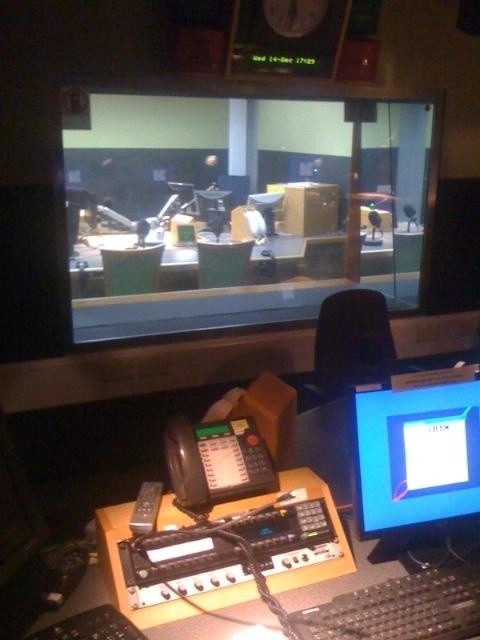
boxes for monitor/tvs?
[344,361,480,572]
[46,61,447,355]
[64,175,292,236]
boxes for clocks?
[224,0,353,82]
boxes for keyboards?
[281,565,480,640]
[23,603,149,640]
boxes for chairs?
[101,234,367,296]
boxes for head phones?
[260,249,276,276]
[77,261,89,287]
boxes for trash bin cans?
[166,414,280,511]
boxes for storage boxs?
[281,180,339,236]
[234,366,299,473]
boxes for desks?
[1,467,479,639]
[68,229,392,303]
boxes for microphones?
[136,221,150,243]
[368,211,383,237]
[403,203,417,227]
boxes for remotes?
[32,548,93,611]
[129,481,164,533]
